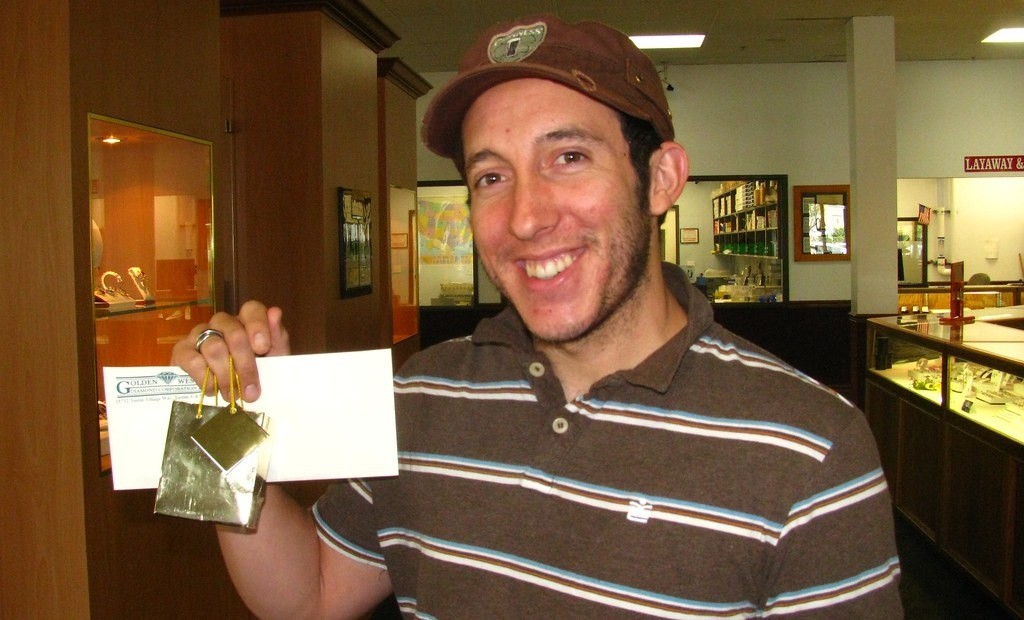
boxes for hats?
[420,13,674,159]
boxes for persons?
[171,16,907,620]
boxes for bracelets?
[101,269,149,291]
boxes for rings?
[196,329,224,353]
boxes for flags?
[918,204,930,224]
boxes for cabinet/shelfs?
[94,298,215,473]
[712,178,780,262]
